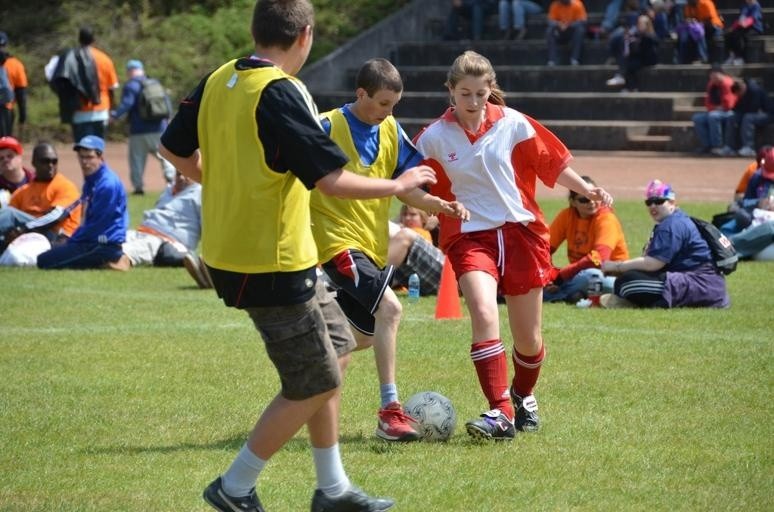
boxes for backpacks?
[135,78,169,121]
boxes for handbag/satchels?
[690,216,739,275]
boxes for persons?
[37,137,127,270]
[544,179,628,304]
[381,204,459,296]
[65,25,119,140]
[1,139,81,268]
[111,58,175,195]
[499,0,774,154]
[1,32,30,139]
[159,3,438,511]
[310,57,470,438]
[183,252,215,289]
[411,49,613,438]
[111,166,203,269]
[715,139,774,264]
[600,180,728,309]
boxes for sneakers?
[184,253,208,289]
[465,407,515,440]
[511,386,540,431]
[608,78,624,85]
[376,402,424,441]
[599,293,633,309]
[198,255,215,288]
[310,487,395,511]
[203,477,263,512]
[712,146,757,156]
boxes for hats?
[0,137,24,155]
[646,180,675,201]
[73,135,104,152]
[762,148,774,179]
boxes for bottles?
[588,273,602,305]
[408,269,420,301]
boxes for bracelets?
[616,260,623,274]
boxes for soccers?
[403,393,455,441]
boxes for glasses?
[645,198,667,207]
[576,197,590,204]
